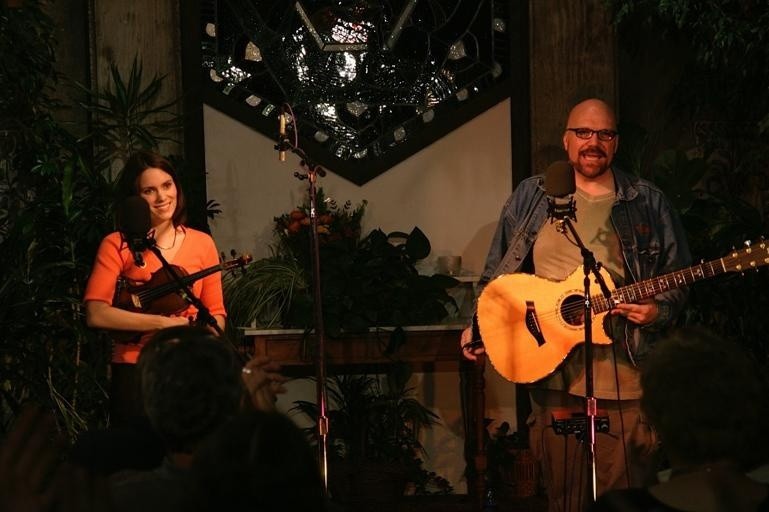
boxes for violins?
[110,254,253,344]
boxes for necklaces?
[153,226,176,250]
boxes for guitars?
[476,233,769,384]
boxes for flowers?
[272,186,368,270]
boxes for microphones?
[119,194,151,254]
[545,159,577,232]
[277,103,290,162]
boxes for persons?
[462,98,692,512]
[584,327,769,512]
[2,324,327,512]
[83,155,227,474]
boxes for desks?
[243,324,495,511]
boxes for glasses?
[567,127,618,141]
